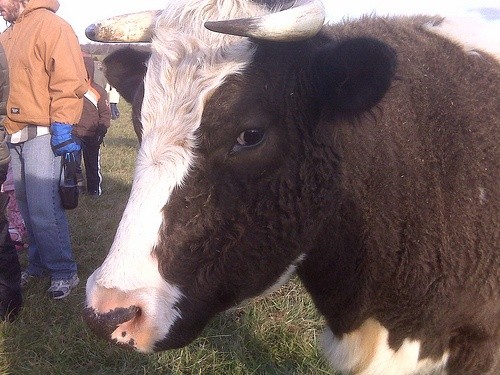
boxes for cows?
[81,0,500,375]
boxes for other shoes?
[88,189,102,196]
[76,180,86,195]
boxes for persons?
[0,43,23,321]
[0,0,89,300]
[71,57,110,198]
[93,56,120,120]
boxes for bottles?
[58,152,79,209]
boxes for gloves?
[109,102,120,120]
[0,165,10,183]
[50,123,81,156]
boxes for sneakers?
[45,273,79,300]
[19,272,37,288]
